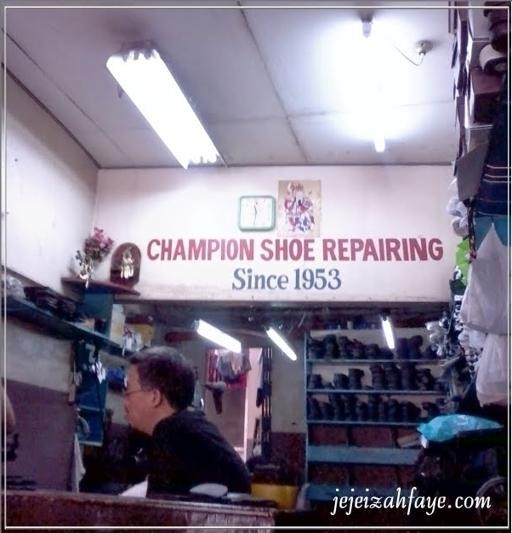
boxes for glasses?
[121,388,152,398]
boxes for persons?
[0,381,16,432]
[124,346,251,506]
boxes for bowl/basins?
[250,481,299,509]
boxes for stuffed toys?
[458,332,482,371]
[425,317,447,357]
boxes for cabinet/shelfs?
[302,329,459,505]
[75,405,106,449]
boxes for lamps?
[105,39,221,172]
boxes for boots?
[307,335,441,421]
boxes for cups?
[189,482,227,499]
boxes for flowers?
[77,225,116,288]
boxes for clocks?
[238,195,276,232]
